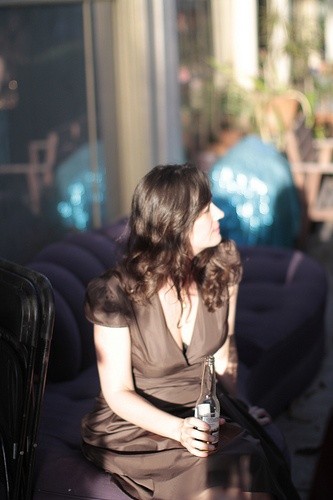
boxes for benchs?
[286,117,333,242]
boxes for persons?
[80,164,301,500]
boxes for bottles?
[194,356,220,453]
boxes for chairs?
[0,258,56,500]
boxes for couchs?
[30,216,329,500]
